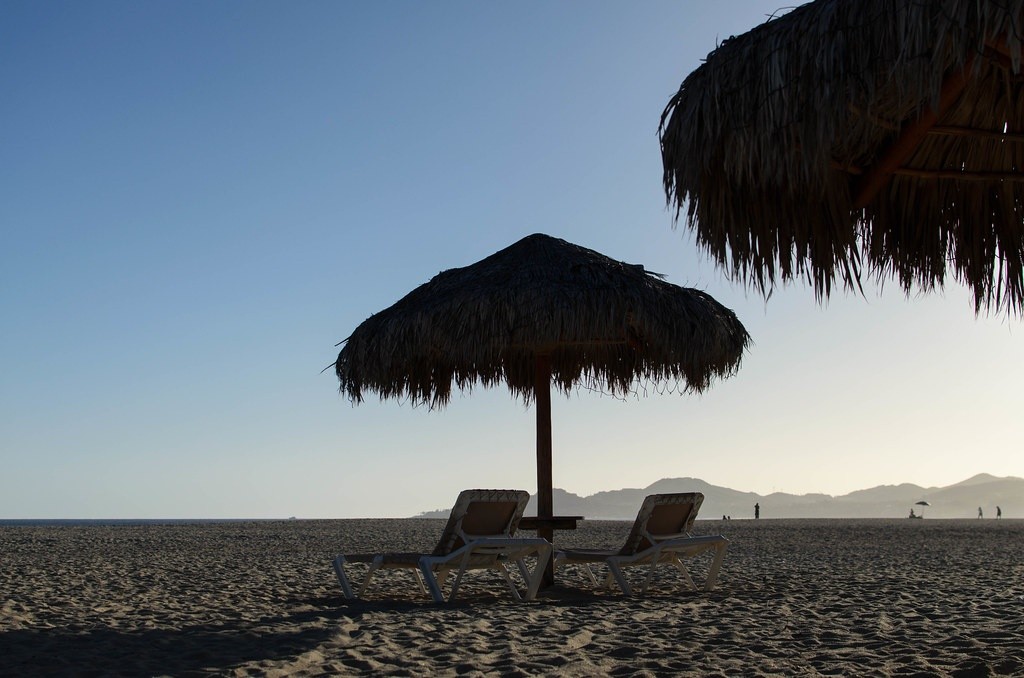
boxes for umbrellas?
[317,231,755,603]
[656,0,1023,322]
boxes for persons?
[995,506,1002,519]
[722,515,726,520]
[977,507,983,519]
[909,509,914,516]
[754,503,760,519]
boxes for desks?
[513,515,586,596]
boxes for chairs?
[527,491,730,596]
[333,489,528,603]
